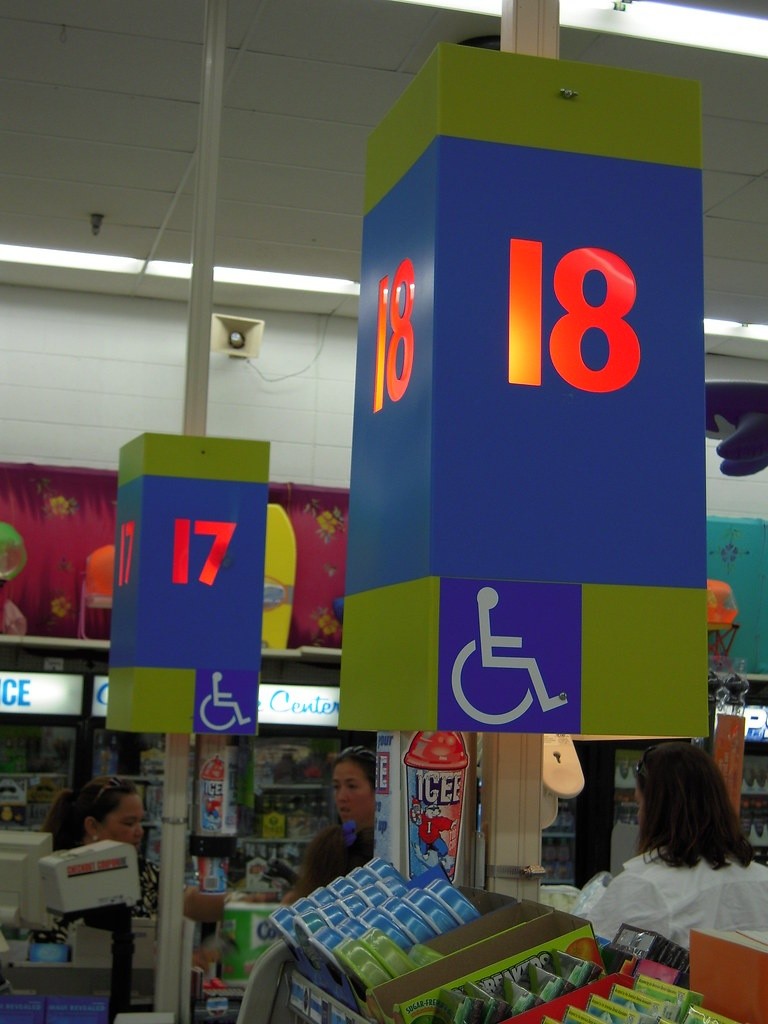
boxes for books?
[689,928,768,1024]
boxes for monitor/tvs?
[0,830,55,931]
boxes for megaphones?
[210,313,264,359]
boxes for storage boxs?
[280,886,640,1024]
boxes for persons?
[34,776,275,1024]
[583,741,768,948]
[282,747,377,904]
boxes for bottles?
[254,793,329,839]
